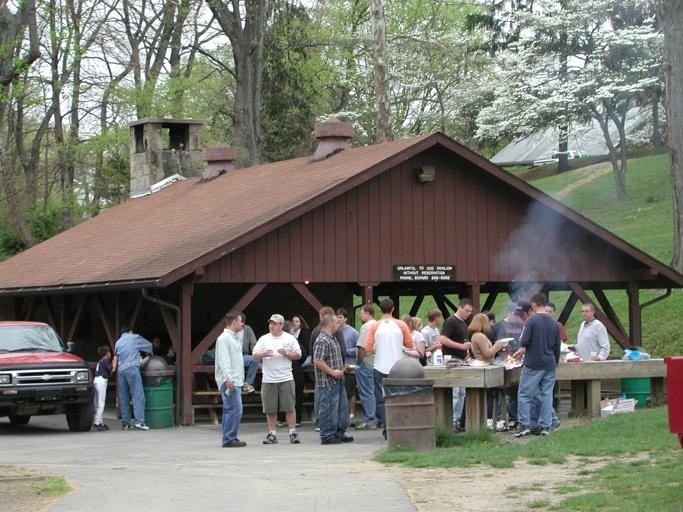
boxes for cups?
[590,352,598,361]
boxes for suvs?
[0,320,93,431]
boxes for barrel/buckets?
[381,376,437,453]
[620,377,652,409]
[140,370,176,430]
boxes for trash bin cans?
[664,355,683,449]
[382,356,437,452]
[621,345,652,409]
[140,355,175,429]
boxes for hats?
[514,300,532,316]
[267,313,285,325]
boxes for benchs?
[190,386,319,425]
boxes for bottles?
[223,380,235,398]
[426,344,444,366]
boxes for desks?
[422,363,506,433]
[552,355,668,420]
[166,362,314,426]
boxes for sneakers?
[93,420,150,431]
[222,438,247,447]
[276,418,562,438]
[289,432,301,444]
[335,434,354,443]
[262,432,278,444]
[320,433,342,444]
